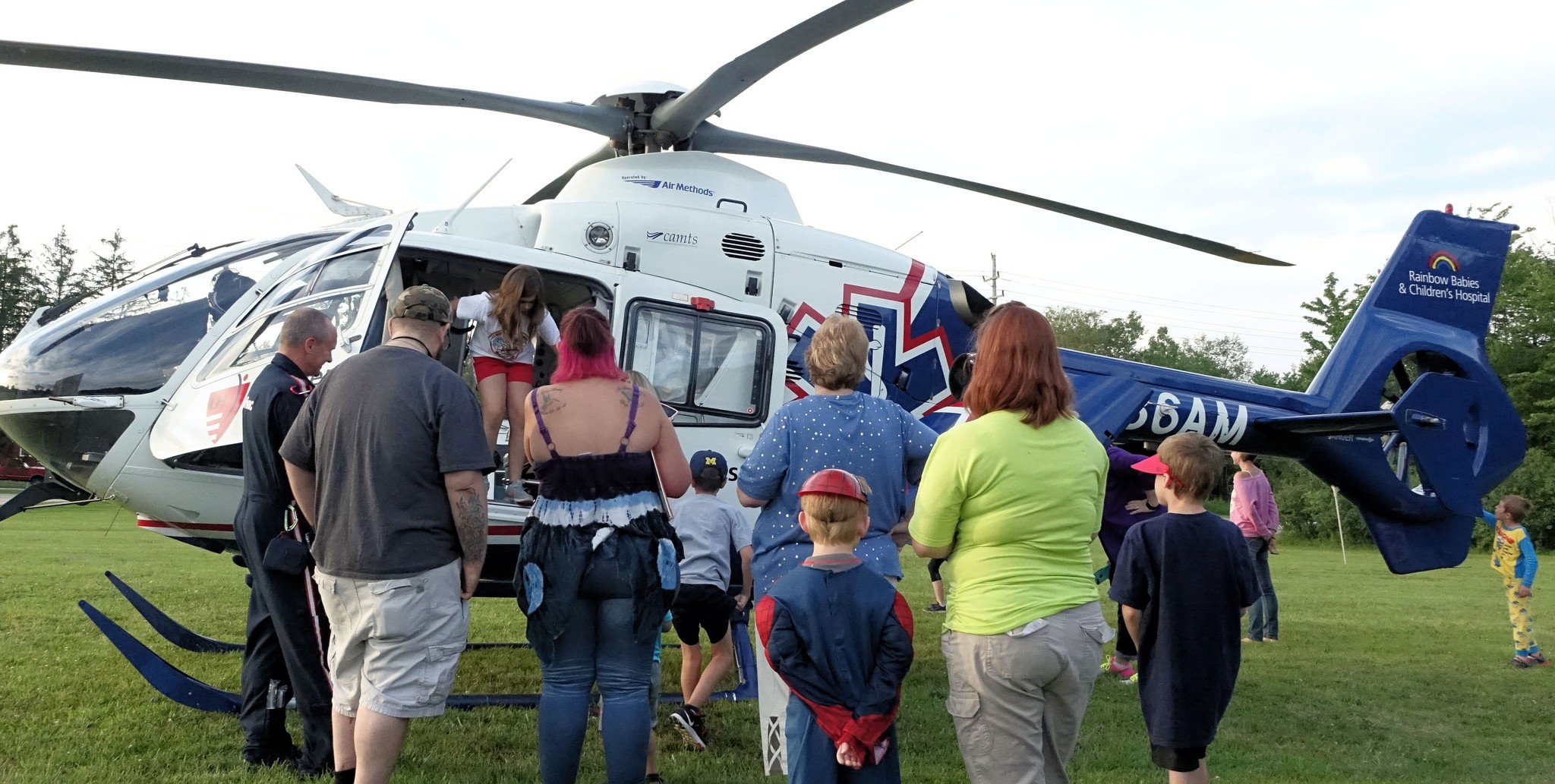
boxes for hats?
[393,284,454,323]
[690,449,728,486]
[1130,453,1183,487]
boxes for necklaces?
[816,387,852,394]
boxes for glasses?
[439,323,450,353]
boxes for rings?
[1137,506,1138,510]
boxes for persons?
[737,313,941,609]
[754,469,914,784]
[1480,494,1549,669]
[450,265,561,505]
[922,558,948,613]
[1098,446,1168,686]
[1230,451,1280,644]
[908,313,1110,784]
[668,450,754,751]
[279,284,498,784]
[1107,431,1262,784]
[233,307,337,779]
[513,306,685,784]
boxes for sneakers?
[1120,673,1139,684]
[668,705,709,750]
[922,601,946,613]
[504,485,533,503]
[1100,654,1134,678]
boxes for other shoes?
[244,745,306,768]
[1503,657,1531,668]
[646,772,665,783]
[295,761,317,779]
[1526,653,1549,666]
[1263,636,1275,642]
[1241,637,1260,643]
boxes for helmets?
[798,469,872,505]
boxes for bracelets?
[1271,537,1276,540]
[1146,500,1161,510]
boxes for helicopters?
[0,0,1530,719]
